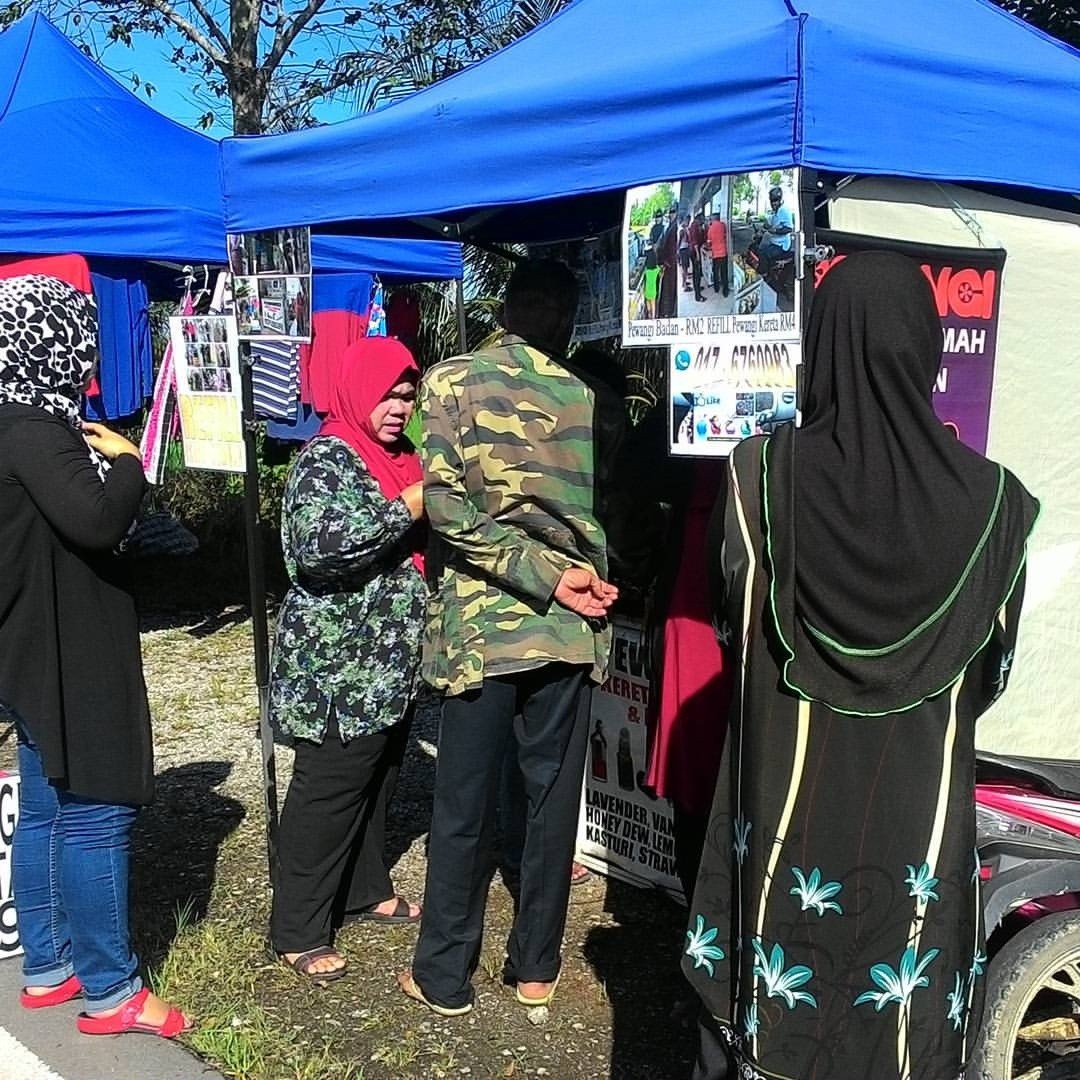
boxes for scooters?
[964,749,1080,1080]
[742,207,796,301]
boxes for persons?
[181,226,307,393]
[248,262,1041,1080]
[0,275,195,1037]
[636,186,792,321]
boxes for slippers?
[517,964,562,1004]
[77,987,195,1038]
[21,975,83,1008]
[284,946,345,981]
[396,968,472,1015]
[570,862,592,883]
[343,896,422,922]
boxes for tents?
[0,10,468,358]
[217,0,1080,880]
